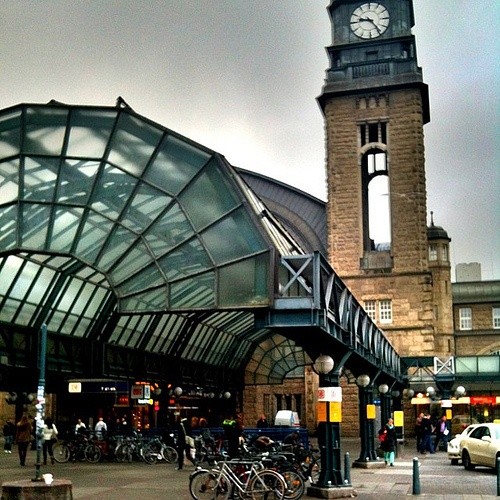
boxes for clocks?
[348,1,392,40]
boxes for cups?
[44,474,52,484]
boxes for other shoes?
[390,463,392,466]
[4,450,8,453]
[385,461,387,464]
[8,450,12,453]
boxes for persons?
[256,414,268,428]
[223,413,240,458]
[415,413,451,454]
[3,414,58,466]
[379,419,398,467]
[175,415,197,470]
[58,416,130,442]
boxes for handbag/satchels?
[185,436,194,447]
[51,434,58,443]
[379,427,387,441]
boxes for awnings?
[470,395,500,408]
[410,396,471,405]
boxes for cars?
[446,422,483,466]
[451,414,485,435]
[457,423,500,475]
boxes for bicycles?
[51,427,322,500]
